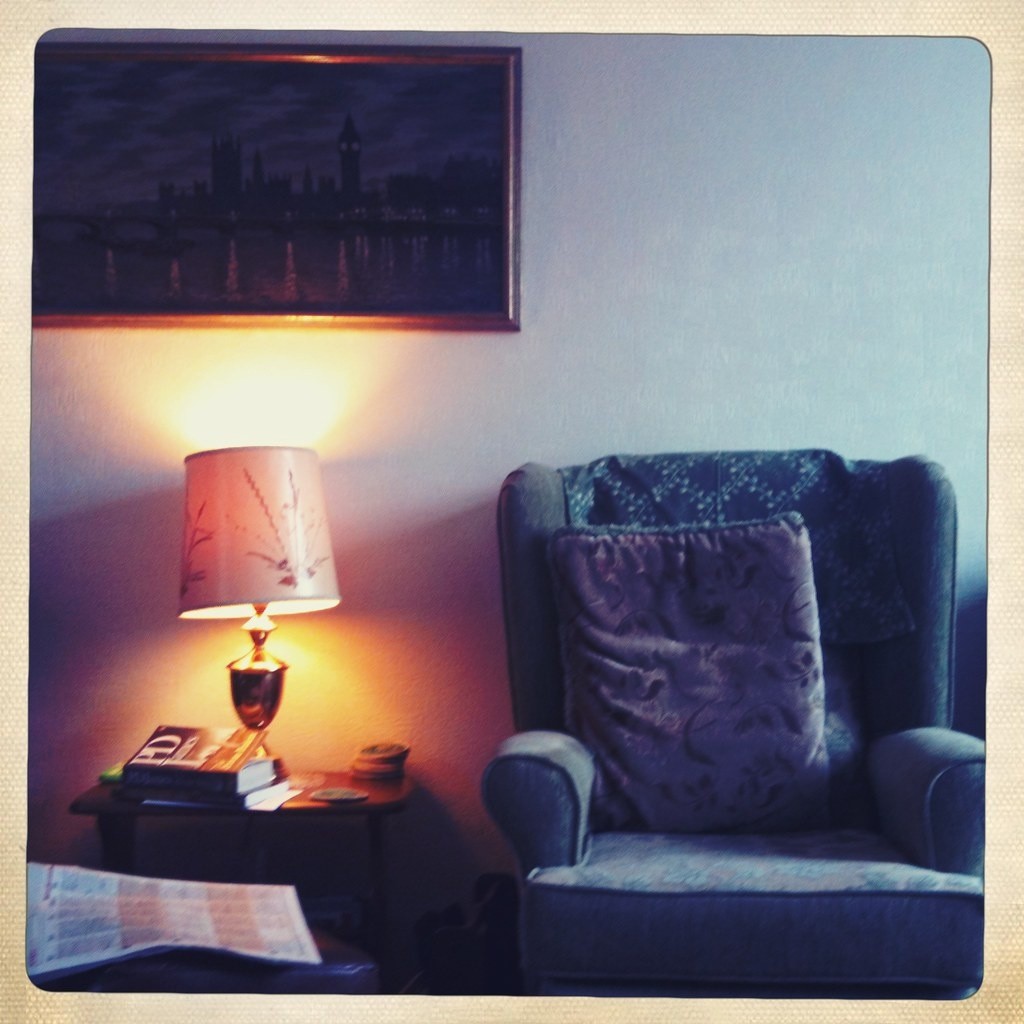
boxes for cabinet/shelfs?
[66,768,417,952]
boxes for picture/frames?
[32,42,521,334]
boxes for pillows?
[546,510,833,834]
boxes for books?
[123,724,304,812]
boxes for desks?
[38,926,386,995]
[478,448,984,998]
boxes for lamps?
[177,446,342,780]
